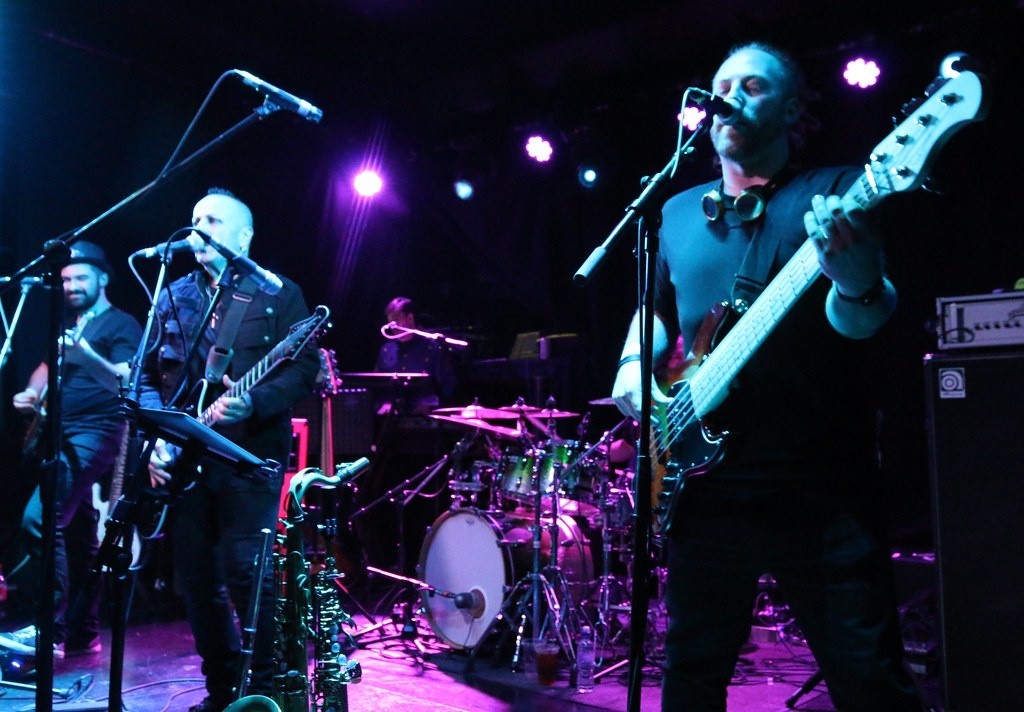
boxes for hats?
[69,241,116,281]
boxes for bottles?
[576,626,595,694]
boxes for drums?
[534,440,607,516]
[451,460,499,511]
[419,506,594,652]
[497,443,541,506]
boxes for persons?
[608,41,926,711]
[0,240,144,661]
[124,188,321,712]
[374,295,460,419]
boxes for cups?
[535,644,560,687]
[521,638,546,680]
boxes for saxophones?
[220,458,371,712]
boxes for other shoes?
[188,694,220,712]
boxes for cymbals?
[427,401,582,437]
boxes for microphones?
[0,276,42,284]
[233,70,323,125]
[687,86,742,125]
[135,234,206,262]
[195,228,283,295]
[455,593,480,608]
[383,321,396,330]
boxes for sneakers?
[50,635,101,659]
[0,626,65,659]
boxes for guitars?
[20,312,97,456]
[648,54,994,547]
[137,304,333,540]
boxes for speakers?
[923,347,1024,712]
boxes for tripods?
[351,566,458,661]
[465,413,668,692]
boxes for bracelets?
[617,353,640,369]
[26,387,39,401]
[835,274,887,306]
